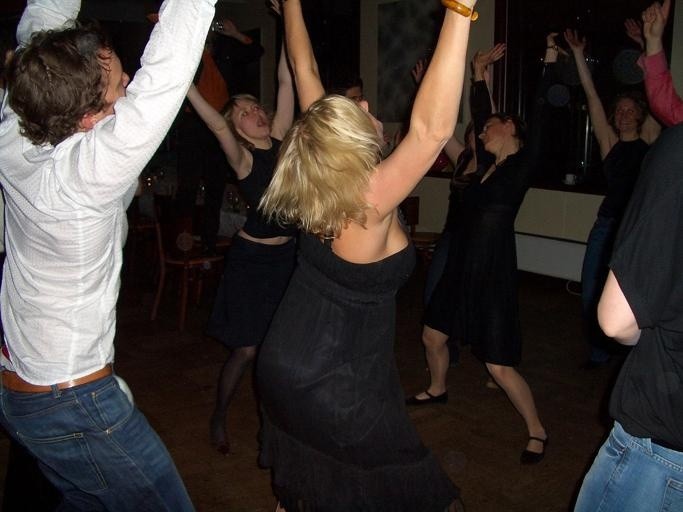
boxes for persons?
[404,31,560,465]
[635,0,683,128]
[561,17,660,415]
[1,0,221,512]
[250,0,475,512]
[408,50,503,390]
[186,0,299,468]
[334,73,365,105]
[185,12,265,257]
[570,115,683,512]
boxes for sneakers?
[404,390,448,405]
[519,435,548,463]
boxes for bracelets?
[545,43,560,50]
[442,0,481,24]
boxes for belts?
[2,362,112,393]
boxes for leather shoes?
[212,426,229,455]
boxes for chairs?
[127,169,234,323]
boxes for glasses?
[349,95,365,102]
